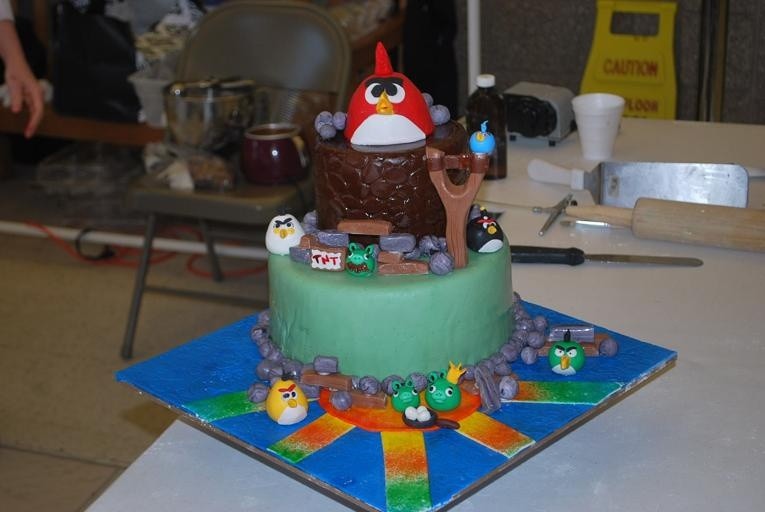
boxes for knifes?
[509,244,703,267]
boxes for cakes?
[248,40,618,430]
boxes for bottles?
[464,75,509,180]
[243,123,310,184]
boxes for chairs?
[116,2,357,370]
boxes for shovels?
[526,158,748,209]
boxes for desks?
[77,110,765,512]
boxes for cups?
[161,82,251,154]
[569,93,625,162]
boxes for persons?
[0,0,45,138]
